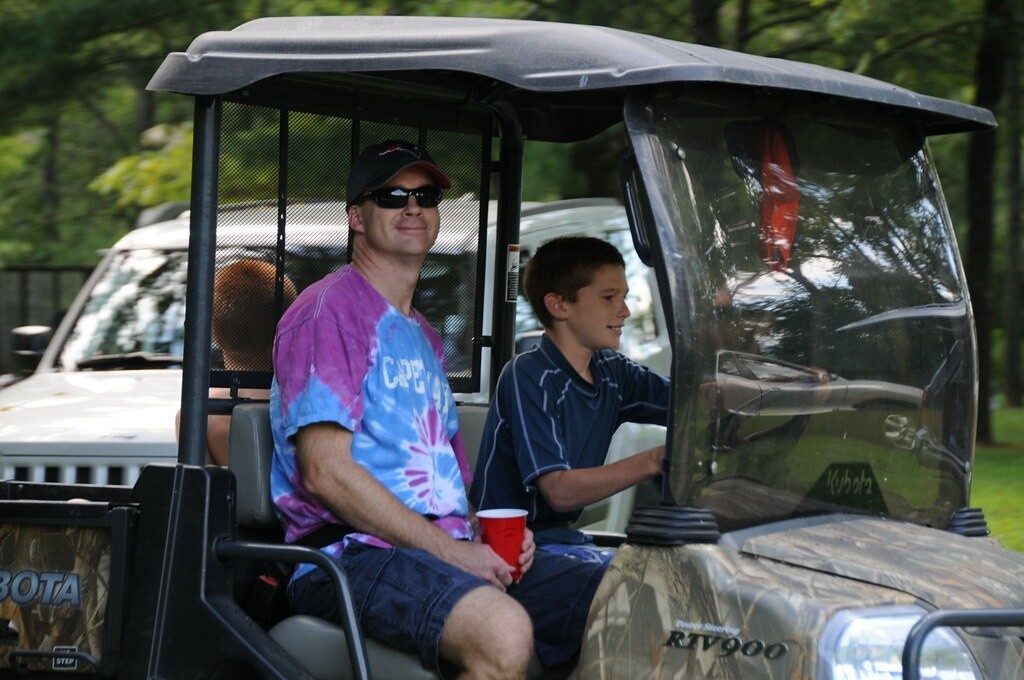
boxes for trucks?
[1,16,1024,680]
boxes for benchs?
[229,401,493,680]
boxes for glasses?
[361,185,443,208]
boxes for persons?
[468,237,831,680]
[174,260,298,468]
[267,141,534,680]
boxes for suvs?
[0,195,727,543]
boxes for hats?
[346,139,451,207]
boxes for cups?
[475,508,529,580]
